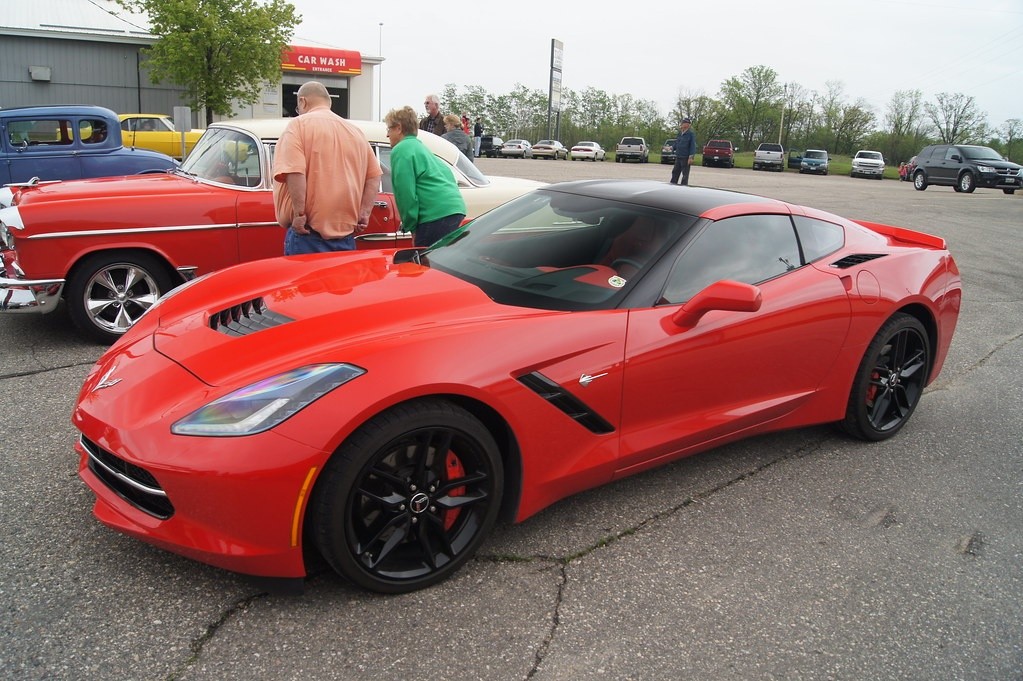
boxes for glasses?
[386,125,398,133]
[295,100,303,114]
[424,102,433,105]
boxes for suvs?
[661,139,677,164]
[909,143,1023,194]
[851,150,888,180]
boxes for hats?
[682,118,690,123]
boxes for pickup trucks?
[752,143,787,172]
[702,140,739,168]
[616,137,650,163]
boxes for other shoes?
[475,156,480,158]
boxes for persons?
[461,112,470,137]
[663,118,696,186]
[385,106,466,248]
[420,96,447,136]
[273,81,379,256]
[441,114,474,163]
[474,118,484,158]
[141,121,154,130]
[898,161,915,182]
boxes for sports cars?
[71,178,964,597]
[0,118,588,349]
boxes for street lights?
[378,23,384,122]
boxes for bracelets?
[294,211,305,218]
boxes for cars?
[571,141,606,162]
[501,139,533,159]
[56,114,254,169]
[788,148,832,175]
[906,156,916,182]
[0,105,182,188]
[531,140,568,160]
[479,135,504,158]
